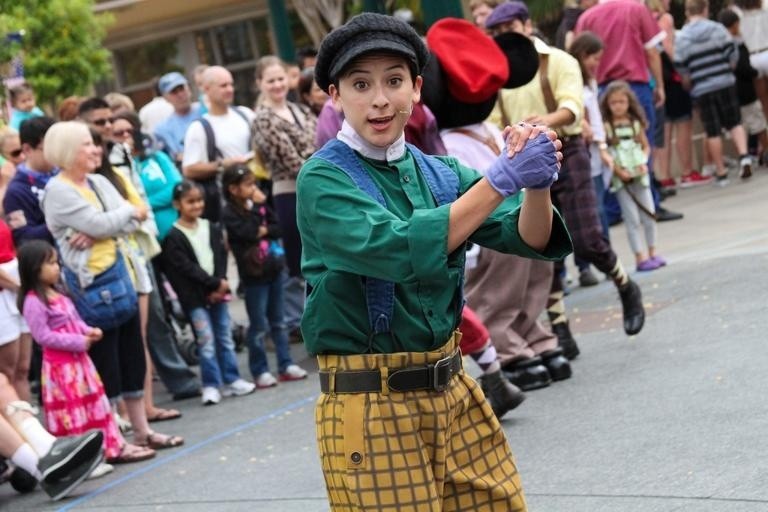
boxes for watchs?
[215,158,225,172]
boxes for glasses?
[1,148,22,157]
[111,130,135,137]
[88,117,113,126]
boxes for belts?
[318,347,463,394]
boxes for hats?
[159,73,189,95]
[316,14,427,93]
[487,2,530,27]
[428,18,510,104]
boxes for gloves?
[486,125,560,198]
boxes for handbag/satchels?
[63,179,139,329]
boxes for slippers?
[650,257,665,266]
[636,259,657,271]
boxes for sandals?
[134,431,184,450]
[107,442,156,464]
[146,409,181,422]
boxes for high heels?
[759,149,768,165]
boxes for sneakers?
[558,273,571,294]
[680,170,713,188]
[659,190,666,200]
[655,209,683,222]
[37,429,104,484]
[174,386,204,400]
[113,414,132,433]
[86,464,113,478]
[739,157,752,178]
[280,365,307,381]
[222,379,256,398]
[715,173,728,185]
[702,164,716,176]
[478,369,525,420]
[202,386,220,406]
[40,447,105,502]
[580,268,597,286]
[255,372,277,389]
[660,179,676,190]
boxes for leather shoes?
[619,278,645,335]
[500,357,552,391]
[552,321,579,361]
[533,347,571,382]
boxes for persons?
[190,64,212,117]
[669,0,753,186]
[669,0,737,179]
[0,162,40,415]
[569,31,613,287]
[297,66,330,117]
[555,0,598,53]
[717,7,767,168]
[181,65,272,218]
[599,79,666,271]
[138,80,175,148]
[0,371,106,501]
[484,1,645,359]
[0,126,26,164]
[296,46,318,72]
[470,1,502,36]
[286,64,300,103]
[8,83,45,131]
[103,92,135,118]
[78,97,200,401]
[220,163,307,388]
[315,97,526,419]
[423,16,571,391]
[161,179,256,406]
[644,0,713,189]
[16,239,125,478]
[112,113,183,245]
[248,55,321,296]
[737,0,768,167]
[43,119,183,463]
[530,28,607,294]
[574,0,683,226]
[153,71,248,296]
[2,116,135,437]
[87,125,180,422]
[297,12,563,512]
